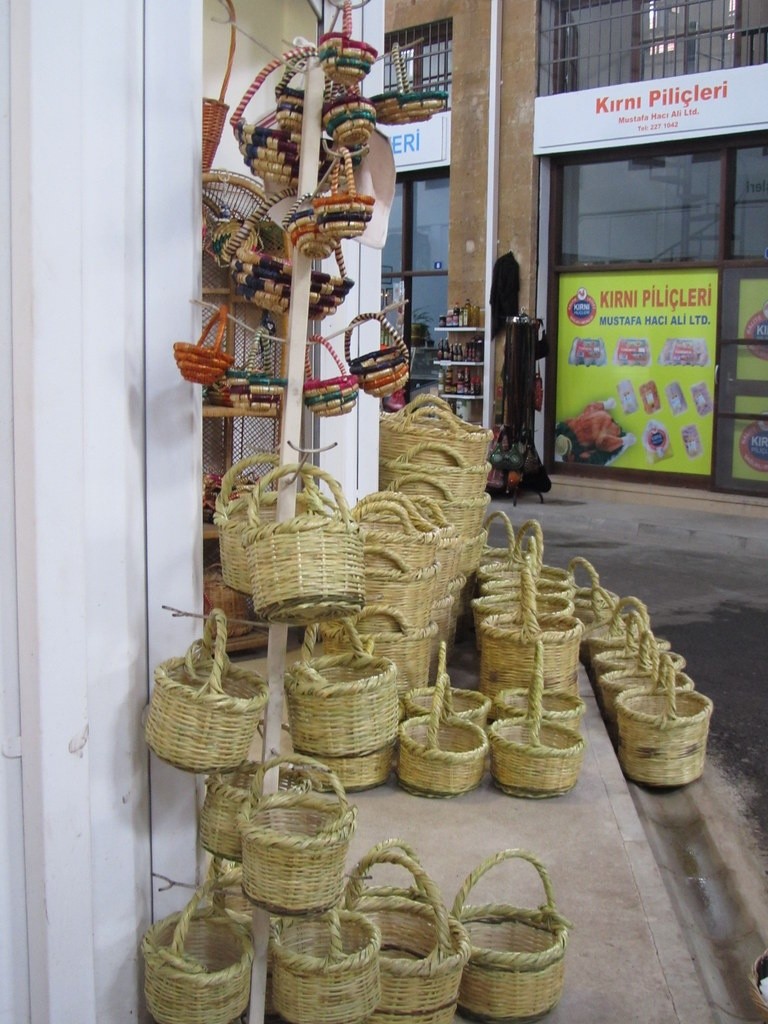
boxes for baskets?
[175,303,236,386]
[141,392,713,1024]
[303,334,360,417]
[173,0,379,321]
[370,42,449,126]
[205,327,287,411]
[345,313,410,398]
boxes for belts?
[502,314,540,449]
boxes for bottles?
[437,299,483,395]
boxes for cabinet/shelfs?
[431,324,484,428]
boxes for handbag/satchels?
[483,424,552,494]
[534,318,550,361]
[534,372,543,411]
